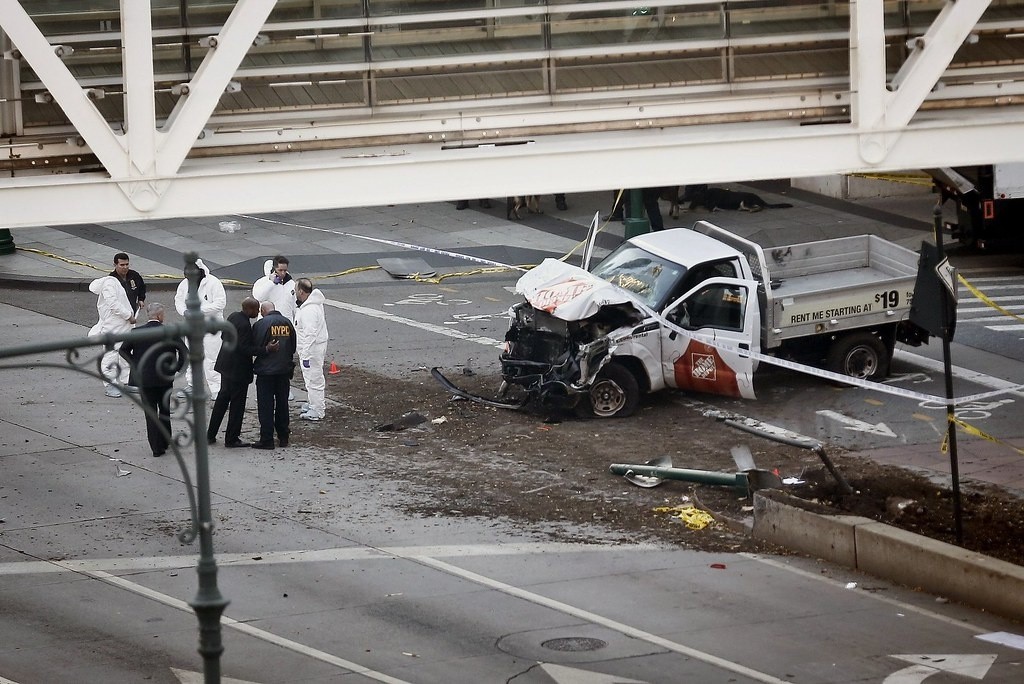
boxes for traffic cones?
[330,360,340,375]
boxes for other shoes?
[208,438,217,445]
[153,447,165,457]
[300,410,325,420]
[125,384,140,393]
[601,214,624,221]
[104,385,122,398]
[301,403,310,412]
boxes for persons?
[252,300,296,448]
[251,255,298,400]
[602,190,624,221]
[642,186,680,231]
[206,298,279,447]
[456,199,491,210]
[552,193,567,210]
[119,303,190,458]
[86,253,147,398]
[294,278,328,420]
[174,258,226,400]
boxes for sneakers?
[177,385,193,397]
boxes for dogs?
[506,195,543,220]
[689,187,793,212]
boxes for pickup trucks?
[500,209,960,418]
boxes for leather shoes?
[225,437,250,447]
[251,441,275,449]
[276,435,289,446]
